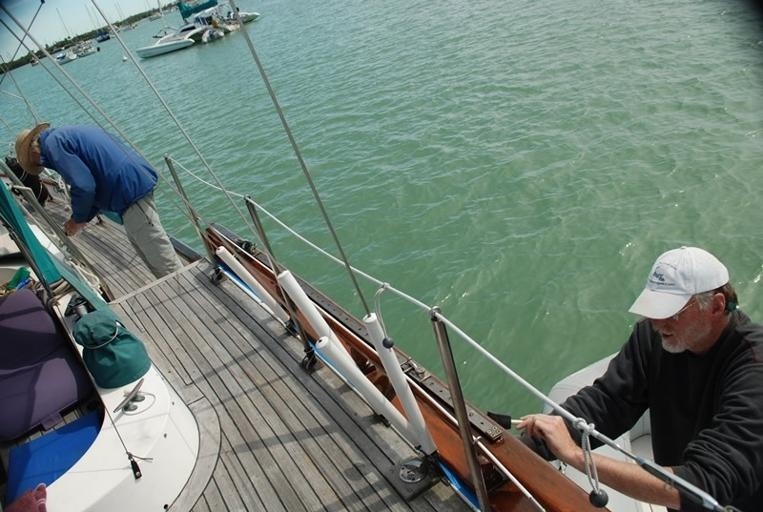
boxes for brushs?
[487,412,525,429]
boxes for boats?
[542,351,668,512]
[31,33,111,66]
[136,7,260,62]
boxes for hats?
[14,121,51,176]
[628,245,730,321]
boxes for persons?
[12,119,186,279]
[482,243,762,512]
